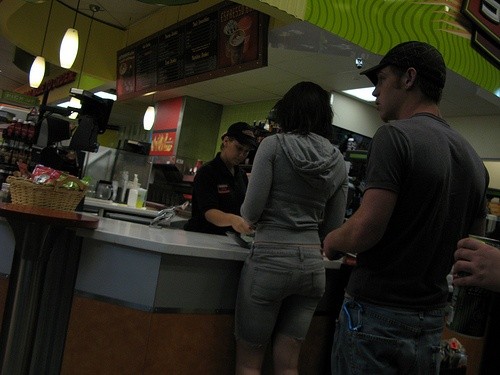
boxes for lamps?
[143,93,156,130]
[68,5,100,120]
[59,0,79,70]
[28,0,53,88]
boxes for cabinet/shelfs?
[0,121,38,203]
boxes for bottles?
[0,142,33,166]
[128,175,141,207]
[8,117,35,140]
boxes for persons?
[184,121,258,236]
[322,40,491,375]
[450,238,500,291]
[235,81,348,375]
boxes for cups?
[136,188,148,208]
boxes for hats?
[360,41,446,86]
[227,122,256,146]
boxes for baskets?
[6,175,85,211]
